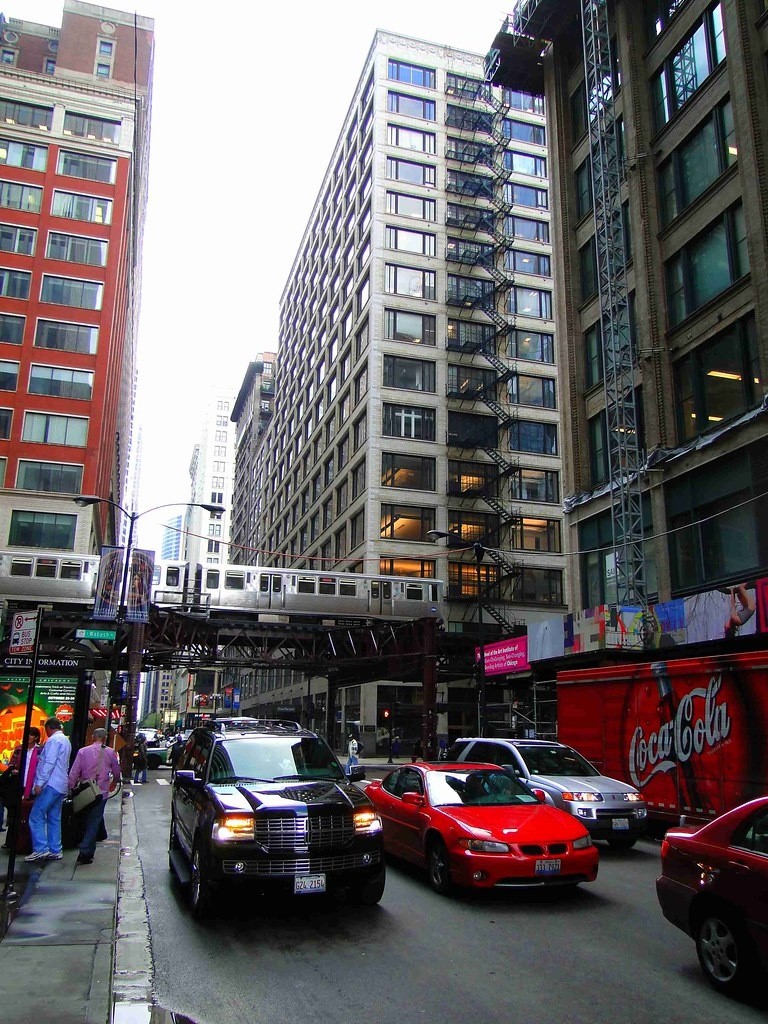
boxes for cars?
[656,796,768,990]
[365,760,601,890]
[137,727,193,747]
[133,740,188,770]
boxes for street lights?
[74,495,225,745]
[210,693,222,717]
[426,530,486,736]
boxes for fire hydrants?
[411,755,417,764]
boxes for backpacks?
[132,743,143,764]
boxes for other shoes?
[77,852,94,862]
[142,780,149,783]
[24,851,63,861]
[134,780,141,783]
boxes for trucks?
[557,648,768,834]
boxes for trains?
[0,551,444,625]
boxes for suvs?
[167,718,392,920]
[444,736,647,851]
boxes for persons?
[392,736,400,759]
[345,734,360,775]
[724,582,755,638]
[24,717,72,861]
[132,572,149,599]
[169,735,185,784]
[2,728,43,849]
[438,735,446,760]
[415,736,421,756]
[133,733,149,783]
[68,727,120,863]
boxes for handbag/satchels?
[352,741,364,755]
[71,778,104,815]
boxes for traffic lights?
[118,726,122,734]
[384,709,390,719]
